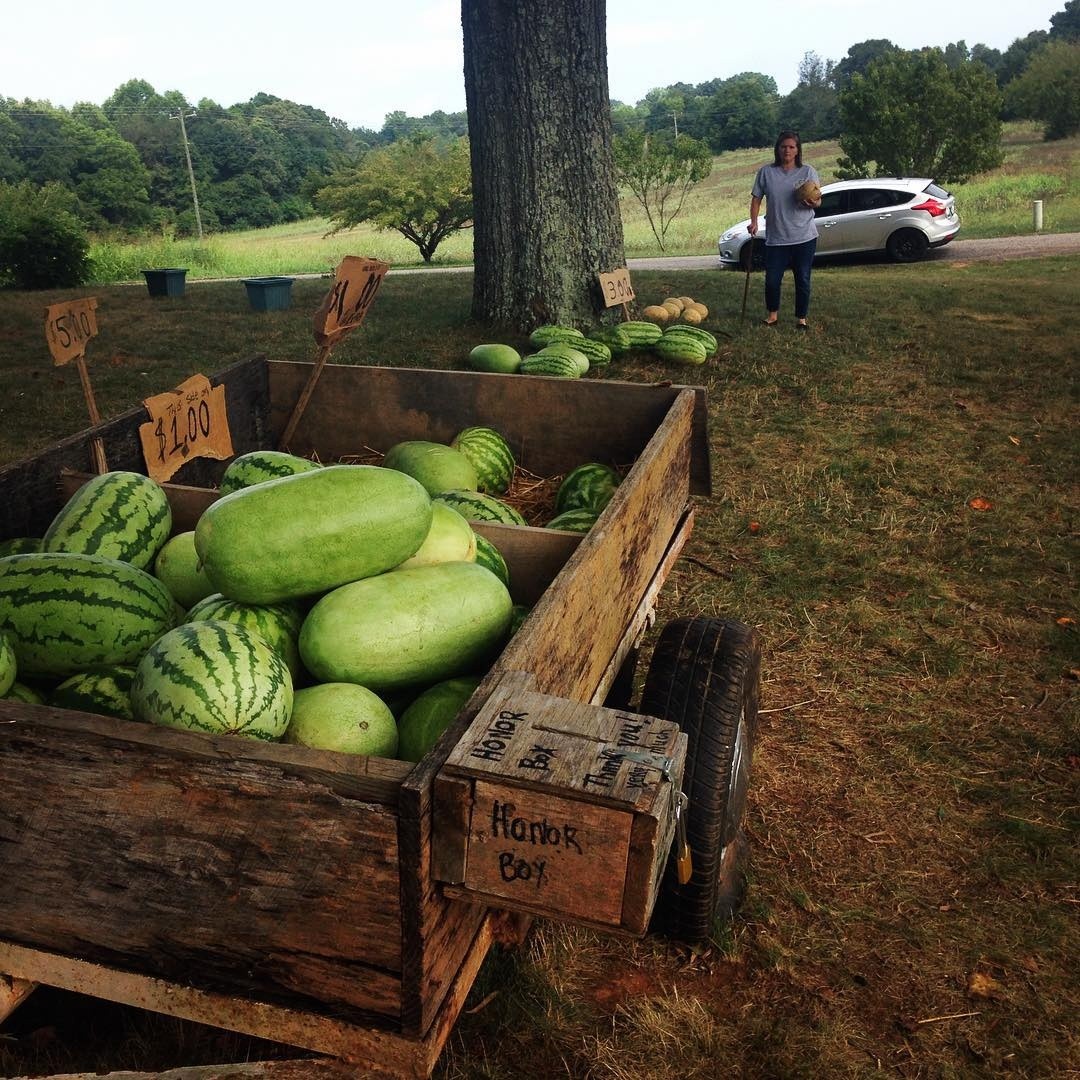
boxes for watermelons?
[0,426,622,760]
[467,321,719,381]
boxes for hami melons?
[642,296,709,324]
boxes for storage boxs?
[243,276,296,313]
[141,268,190,298]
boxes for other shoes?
[799,323,808,331]
[760,320,778,326]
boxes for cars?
[718,176,961,273]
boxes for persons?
[747,131,821,332]
